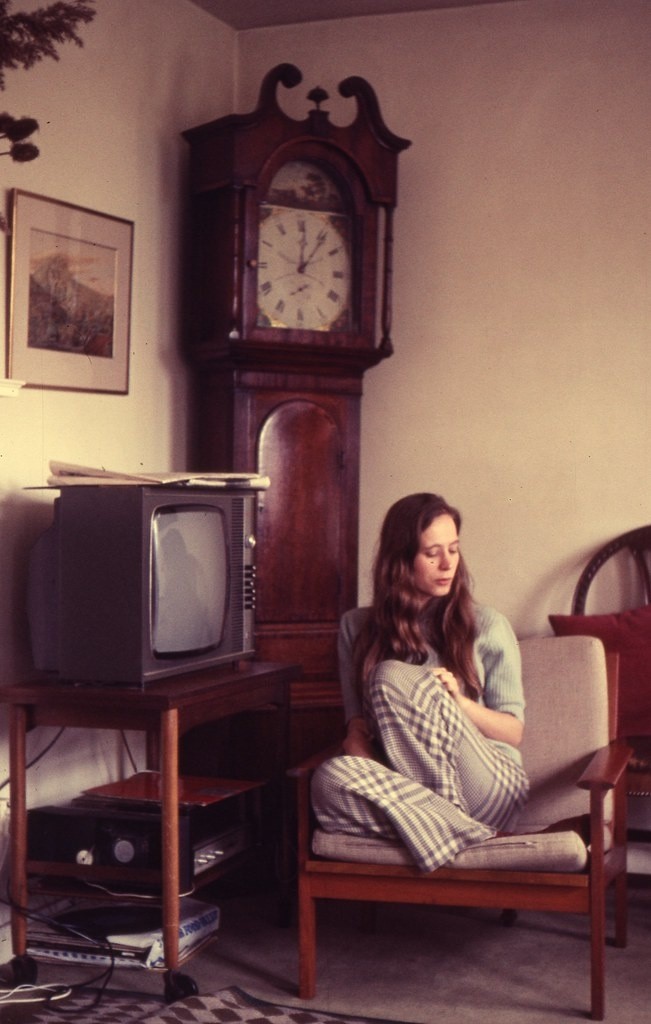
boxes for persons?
[310,489,529,873]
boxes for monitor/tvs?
[27,483,257,689]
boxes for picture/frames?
[8,187,135,395]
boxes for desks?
[0,662,300,1002]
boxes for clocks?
[180,64,415,365]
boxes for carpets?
[0,986,402,1024]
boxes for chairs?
[573,525,651,833]
[286,636,631,1019]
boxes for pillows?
[549,607,651,738]
